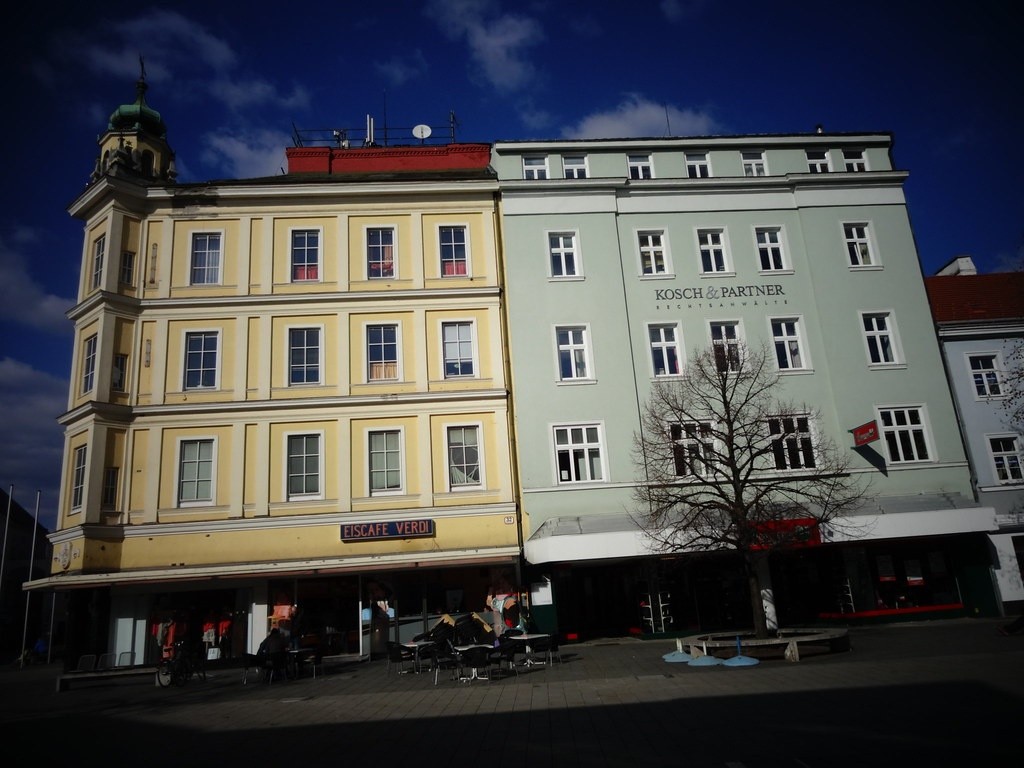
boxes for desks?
[509,634,550,667]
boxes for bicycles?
[154,639,211,689]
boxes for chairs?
[239,651,274,688]
[115,651,135,670]
[68,655,96,673]
[300,646,325,680]
[386,643,418,680]
[431,648,460,686]
[95,653,116,671]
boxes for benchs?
[55,667,158,692]
